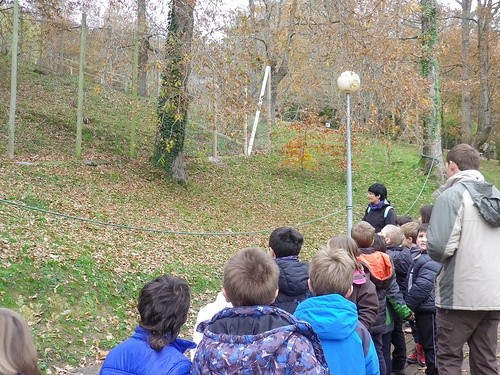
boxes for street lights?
[336,71,360,237]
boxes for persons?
[426,143,500,375]
[0,184,443,375]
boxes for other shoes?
[406,343,418,364]
[405,321,416,326]
[405,327,413,334]
[416,343,425,367]
